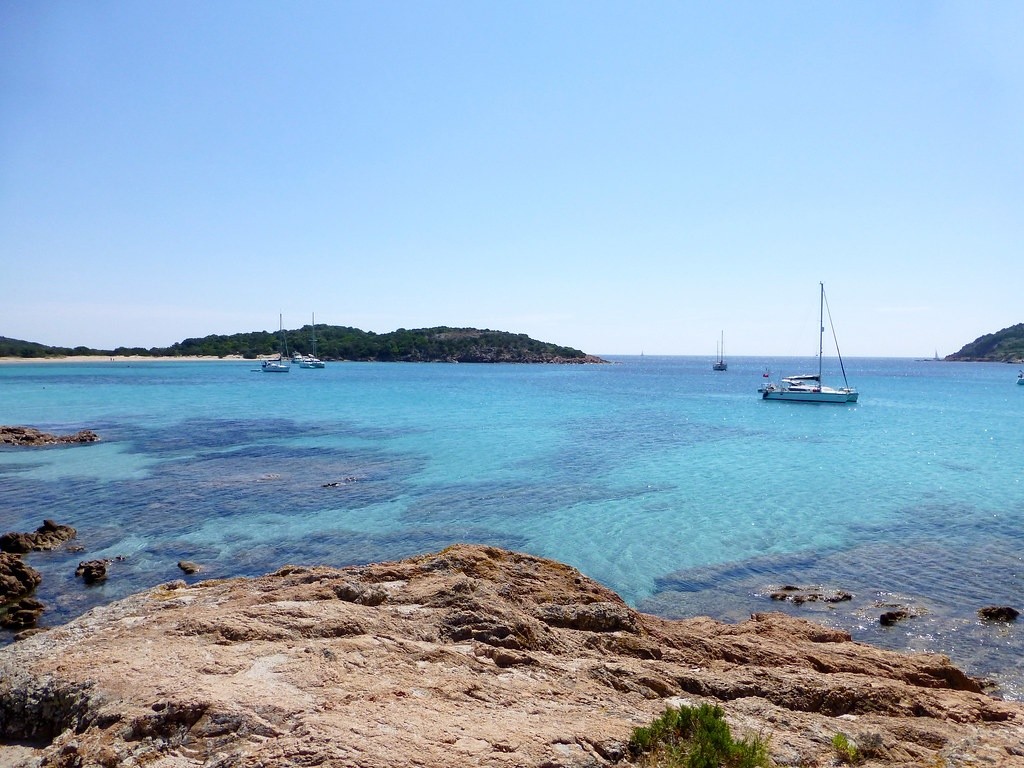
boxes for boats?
[304,354,321,363]
[293,350,303,363]
[256,353,281,363]
[221,354,245,360]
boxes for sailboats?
[299,314,326,369]
[262,313,292,374]
[712,329,728,372]
[756,281,860,405]
